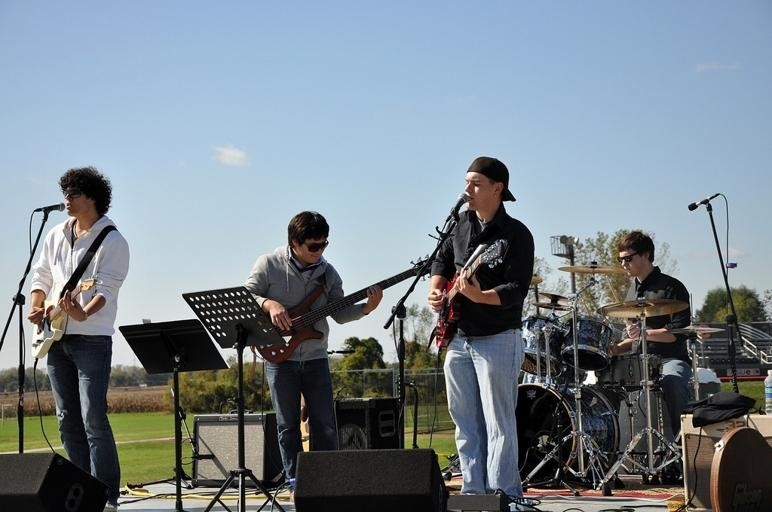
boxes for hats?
[467,157,516,201]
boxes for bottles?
[764,370,772,415]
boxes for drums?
[520,315,565,373]
[560,312,611,370]
[517,383,618,484]
[594,353,662,391]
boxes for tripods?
[520,300,625,496]
[201,468,288,512]
[594,381,684,492]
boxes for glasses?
[63,190,82,199]
[304,239,329,252]
[617,252,637,263]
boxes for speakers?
[0,451,109,512]
[446,492,504,512]
[335,397,405,449]
[294,447,446,512]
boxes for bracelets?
[79,310,88,322]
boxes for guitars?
[33,279,98,360]
[437,239,506,351]
[255,259,432,364]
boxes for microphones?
[446,193,469,221]
[31,203,66,214]
[589,259,597,287]
[687,193,721,212]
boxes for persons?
[243,210,383,491]
[427,156,534,505]
[607,231,691,469]
[27,165,130,511]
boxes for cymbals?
[557,264,626,275]
[597,298,689,317]
[530,273,542,284]
[538,291,567,300]
[533,302,571,312]
